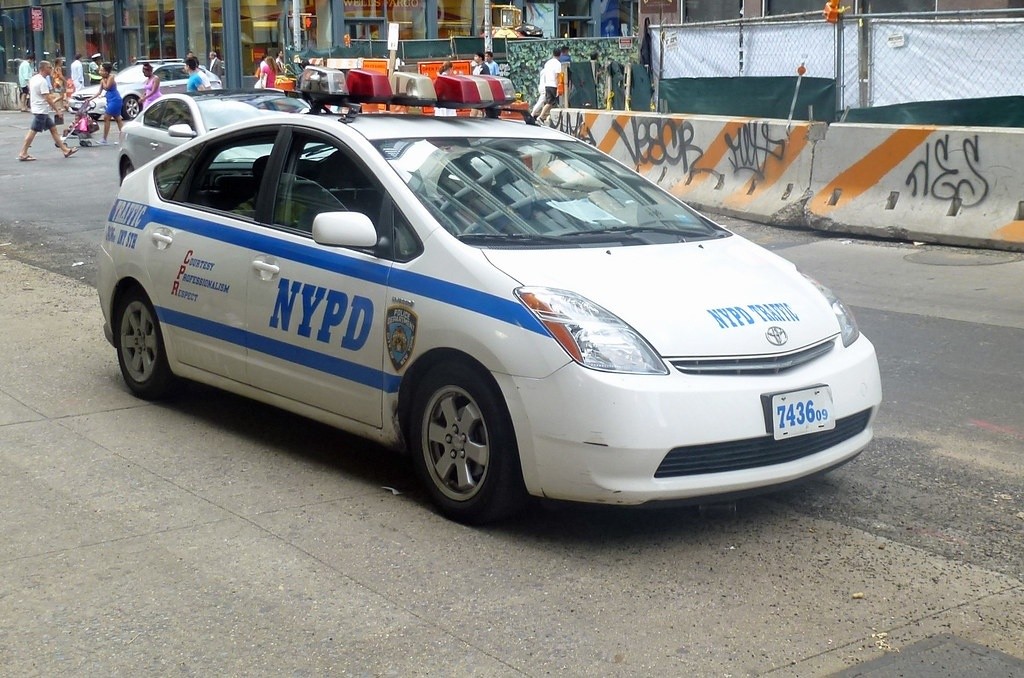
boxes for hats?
[92,53,103,59]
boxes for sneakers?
[536,116,544,124]
[114,142,118,144]
[96,140,107,145]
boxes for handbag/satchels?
[254,79,261,88]
[255,68,261,78]
[556,72,564,97]
[75,116,87,131]
[260,73,267,88]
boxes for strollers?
[54,98,100,148]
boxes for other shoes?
[531,114,536,120]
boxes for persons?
[484,51,500,76]
[233,154,310,219]
[86,63,124,145]
[71,53,84,94]
[259,50,284,89]
[18,60,77,161]
[208,51,222,81]
[182,51,212,92]
[434,60,458,117]
[137,62,163,105]
[589,52,625,89]
[469,50,490,118]
[531,46,571,125]
[19,55,34,113]
[51,57,77,113]
[88,52,103,85]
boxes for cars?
[1,49,96,86]
[68,57,223,121]
[96,66,884,528]
[117,87,341,192]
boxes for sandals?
[64,148,79,157]
[19,155,36,161]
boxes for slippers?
[21,108,30,112]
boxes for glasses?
[47,67,52,69]
